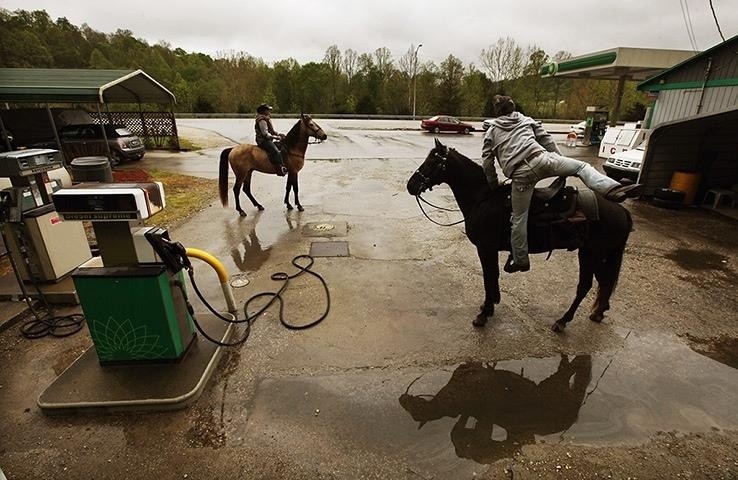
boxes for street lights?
[413,44,423,121]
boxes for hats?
[256,103,272,112]
[492,94,515,116]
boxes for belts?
[517,150,542,167]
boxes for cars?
[570,120,608,135]
[17,124,145,166]
[420,115,475,134]
[602,139,649,182]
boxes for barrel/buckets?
[668,168,701,206]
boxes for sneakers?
[278,168,288,175]
[606,184,646,202]
[505,259,530,272]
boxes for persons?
[256,103,290,176]
[482,93,646,273]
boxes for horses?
[217,111,327,218]
[406,136,632,335]
